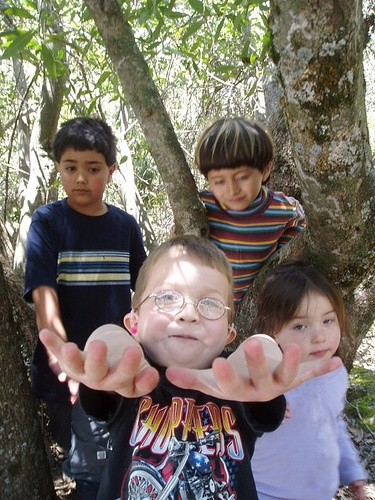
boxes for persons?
[21,116,139,500]
[37,233,344,500]
[170,117,308,318]
[247,259,375,500]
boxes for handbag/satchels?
[61,396,121,483]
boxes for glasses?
[133,289,233,322]
